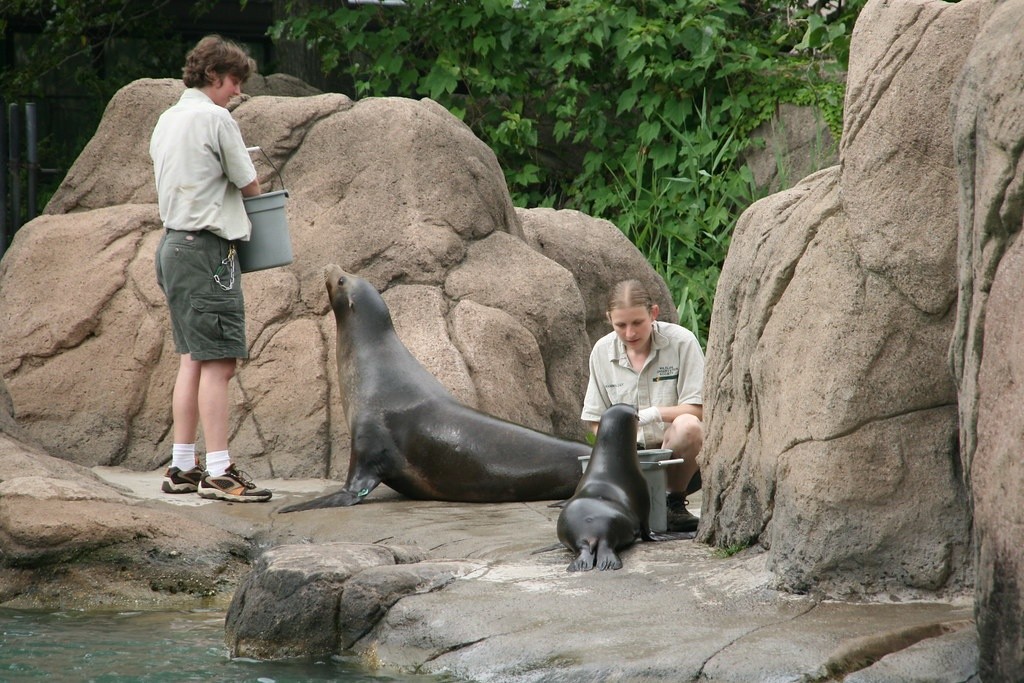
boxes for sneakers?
[666,491,699,532]
[198,462,272,502]
[162,464,206,493]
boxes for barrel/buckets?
[577,448,684,534]
[235,146,293,274]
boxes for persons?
[150,35,274,502]
[580,278,704,532]
